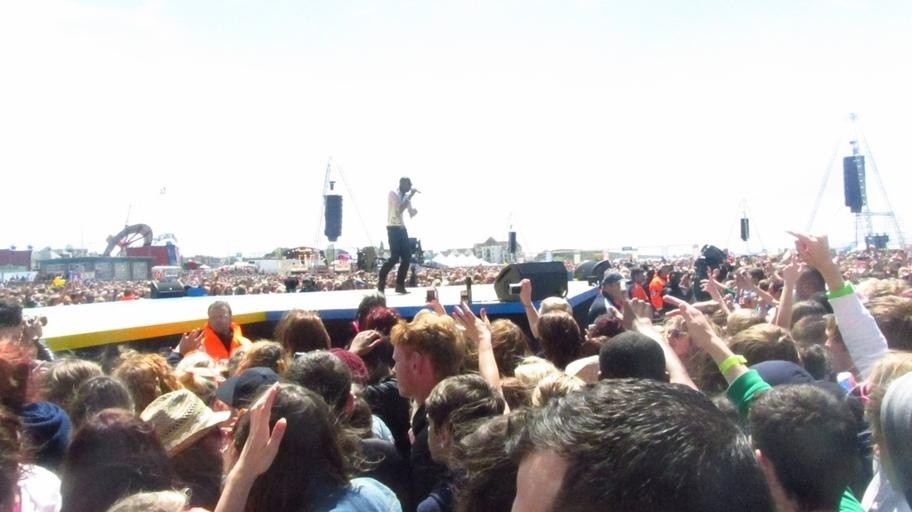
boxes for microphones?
[409,187,422,194]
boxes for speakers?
[324,194,343,242]
[575,259,611,281]
[740,218,750,240]
[843,156,866,213]
[151,279,184,298]
[508,231,518,252]
[494,262,569,301]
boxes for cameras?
[25,312,48,332]
[509,284,522,296]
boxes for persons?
[378,176,417,294]
[0,249,912,511]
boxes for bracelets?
[405,198,410,202]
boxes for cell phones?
[427,290,435,303]
[460,290,469,306]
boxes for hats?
[217,366,287,410]
[141,389,231,458]
